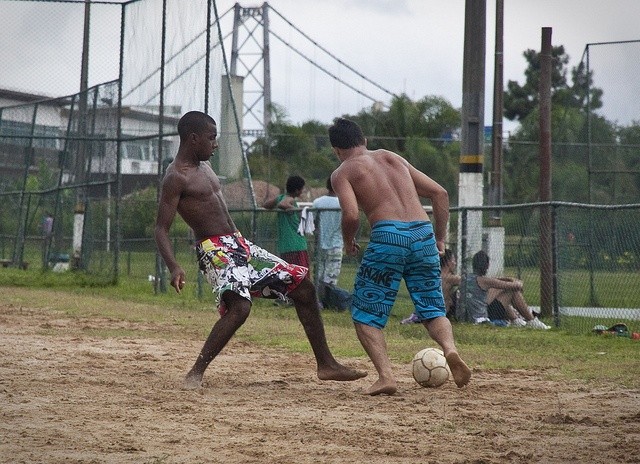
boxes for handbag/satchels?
[325,281,352,310]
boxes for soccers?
[411,347,450,387]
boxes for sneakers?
[511,318,526,327]
[524,316,552,330]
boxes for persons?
[263,176,310,306]
[153,111,368,390]
[330,117,471,395]
[459,250,551,330]
[438,249,461,317]
[38,210,53,265]
[311,178,345,308]
